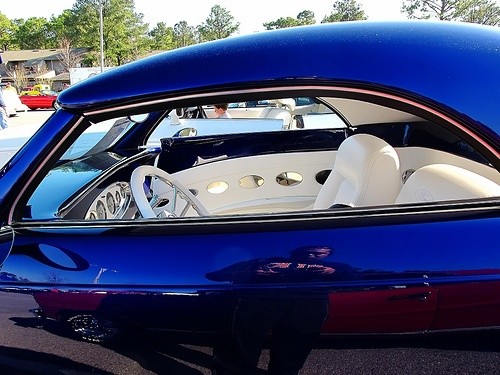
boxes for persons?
[4,82,16,92]
[214,104,232,118]
[63,83,69,89]
[203,245,361,375]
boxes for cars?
[17,90,61,110]
[0,19,499,375]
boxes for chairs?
[313,134,405,208]
[396,163,500,204]
[262,109,291,126]
